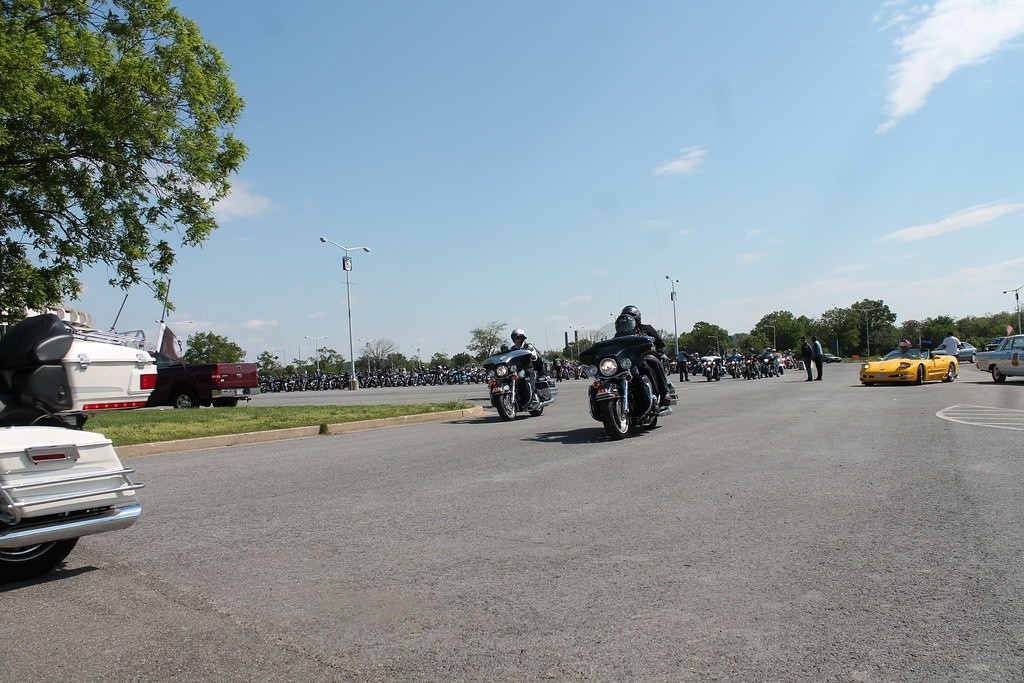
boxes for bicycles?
[1003,285,1024,334]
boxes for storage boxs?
[0,426,137,517]
[32,335,159,416]
[533,355,552,372]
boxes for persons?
[942,331,962,379]
[812,336,824,381]
[259,354,588,389]
[677,347,696,382]
[512,328,544,376]
[978,342,986,351]
[671,347,797,370]
[501,344,509,352]
[801,337,812,382]
[614,306,671,406]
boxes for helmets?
[767,348,772,352]
[772,349,776,352]
[511,328,527,343]
[733,348,738,352]
[712,347,716,353]
[621,305,641,326]
[750,347,754,352]
[615,313,637,332]
[681,346,687,351]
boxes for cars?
[823,354,842,363]
[931,341,978,363]
[974,335,1024,384]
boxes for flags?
[1007,324,1013,335]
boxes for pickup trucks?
[143,352,258,410]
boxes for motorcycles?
[258,362,796,393]
[481,350,559,421]
[578,322,679,440]
[0,313,157,582]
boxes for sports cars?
[858,348,959,387]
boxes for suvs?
[985,335,1006,352]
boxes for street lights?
[320,237,371,390]
[708,336,719,353]
[764,325,776,349]
[305,337,328,377]
[411,346,426,357]
[849,307,881,357]
[666,275,680,354]
[359,340,375,372]
